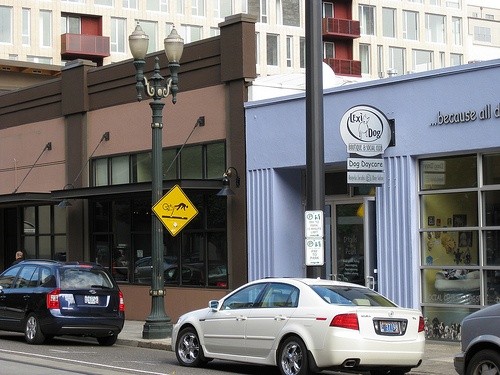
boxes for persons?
[11,251,24,266]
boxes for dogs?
[424,316,463,342]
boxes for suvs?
[0,258,125,347]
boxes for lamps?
[217,166,241,195]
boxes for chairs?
[274,294,293,306]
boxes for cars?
[171,277,426,375]
[133,254,228,288]
[453,301,500,375]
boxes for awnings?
[0,191,61,208]
[51,178,224,200]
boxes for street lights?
[127,22,186,339]
